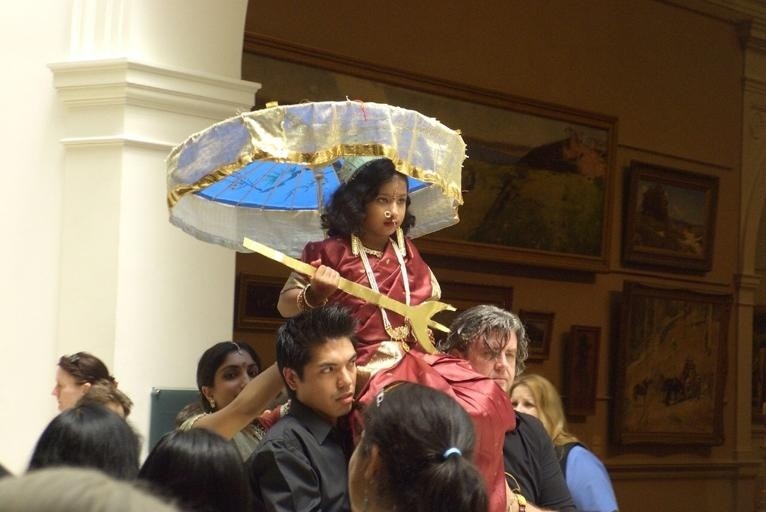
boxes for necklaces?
[354,227,414,353]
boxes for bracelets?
[509,493,528,512]
[296,283,329,316]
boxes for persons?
[641,182,703,255]
[2,304,619,512]
[277,157,508,511]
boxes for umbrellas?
[163,96,469,348]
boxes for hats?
[338,154,389,186]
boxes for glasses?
[375,380,410,408]
